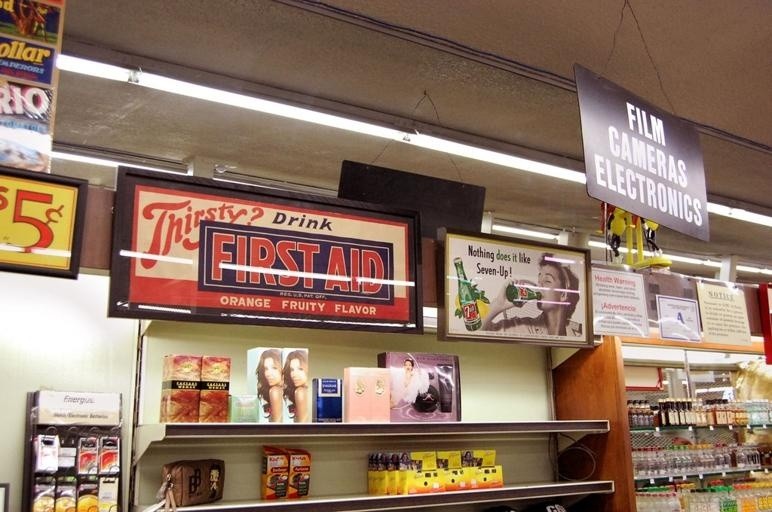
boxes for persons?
[390,359,430,407]
[478,256,582,337]
[255,350,282,424]
[31,3,54,42]
[281,349,310,423]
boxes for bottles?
[506,285,542,302]
[626,394,772,512]
[454,258,483,332]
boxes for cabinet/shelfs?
[551,334,772,510]
[129,414,618,510]
